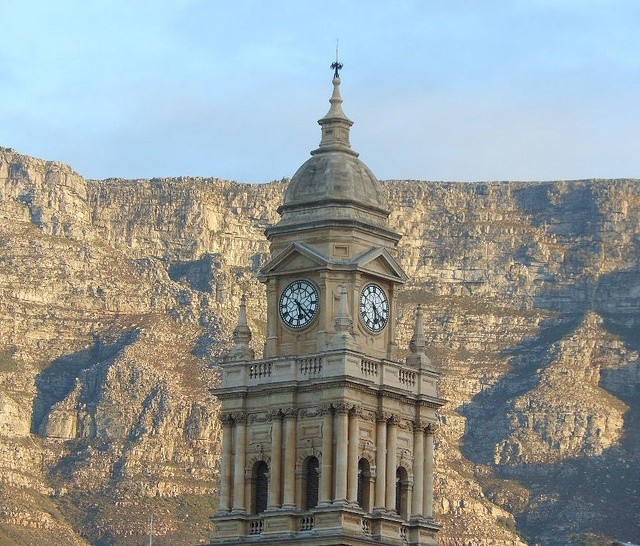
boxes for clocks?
[278,278,320,330]
[358,283,390,334]
